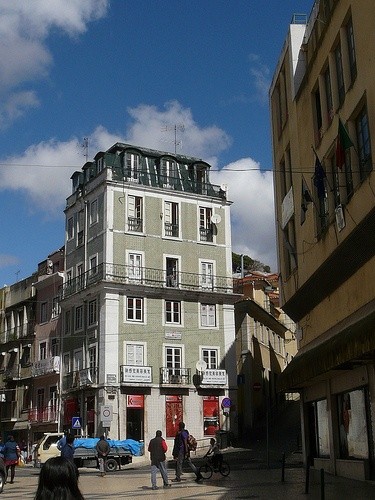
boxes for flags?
[301,178,311,225]
[314,157,327,210]
[336,119,354,171]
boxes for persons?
[0,436,20,484]
[34,457,84,500]
[204,438,223,470]
[57,431,69,451]
[61,433,75,458]
[148,430,171,490]
[171,422,203,482]
[96,436,111,477]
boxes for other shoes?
[195,476,203,481]
[101,472,104,477]
[152,487,159,490]
[172,478,181,482]
[164,484,171,488]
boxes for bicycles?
[198,453,232,480]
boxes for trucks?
[34,432,133,472]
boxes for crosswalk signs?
[72,417,82,429]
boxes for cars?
[0,458,8,492]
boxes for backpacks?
[186,434,198,451]
